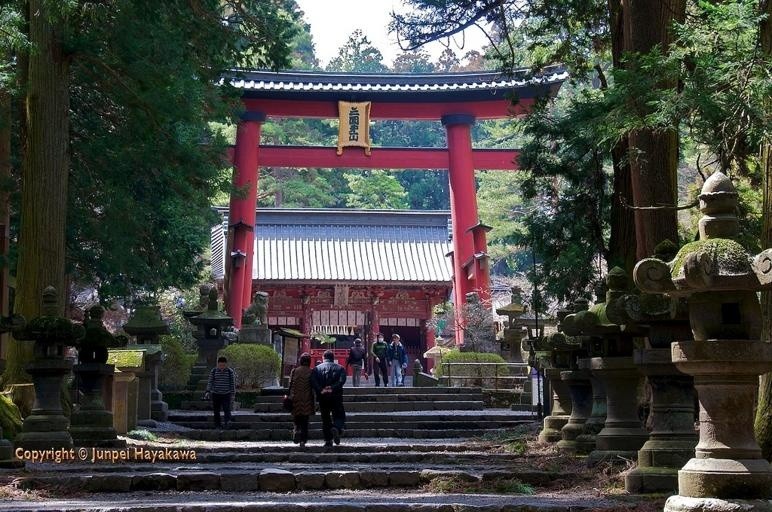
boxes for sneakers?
[224,420,233,426]
[299,442,309,453]
[330,427,341,445]
[370,377,416,386]
[323,440,333,447]
[293,431,300,444]
[217,425,223,434]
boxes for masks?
[378,337,384,343]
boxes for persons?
[312,349,347,446]
[370,333,389,386]
[288,351,315,447]
[345,338,369,387]
[386,334,407,386]
[203,357,235,429]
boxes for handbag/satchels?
[283,398,294,411]
